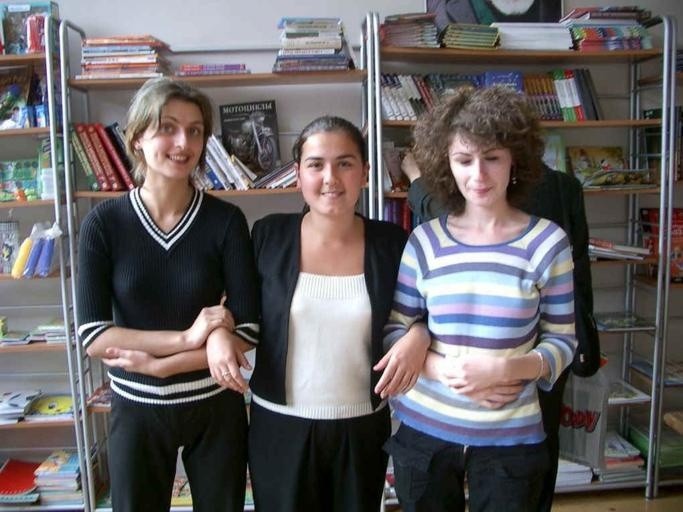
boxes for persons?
[380,84,580,510]
[73,77,260,511]
[401,149,599,511]
[207,116,431,510]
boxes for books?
[2,2,112,510]
[384,467,396,501]
[588,237,681,482]
[382,8,655,191]
[554,456,592,486]
[170,476,192,506]
[77,100,298,190]
[81,18,355,78]
[636,48,681,283]
[384,197,426,236]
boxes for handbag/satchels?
[572,315,600,377]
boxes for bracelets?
[533,348,543,381]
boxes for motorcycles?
[224,107,279,175]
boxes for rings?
[220,371,230,377]
